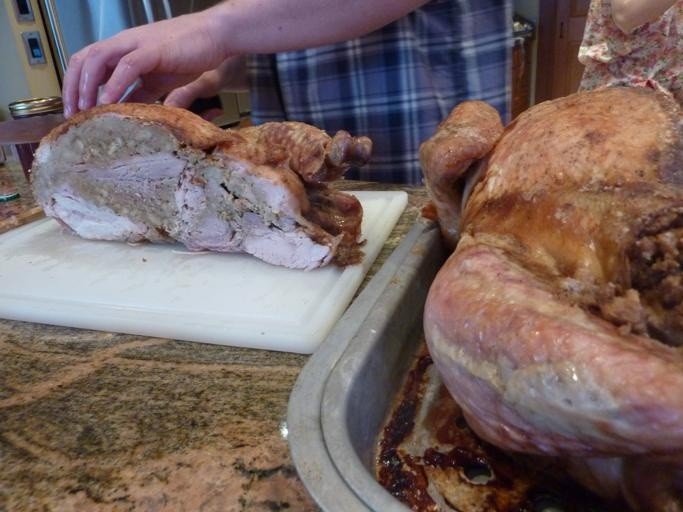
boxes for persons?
[59,0,516,189]
[575,1,683,110]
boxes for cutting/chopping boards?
[0,188,407,353]
